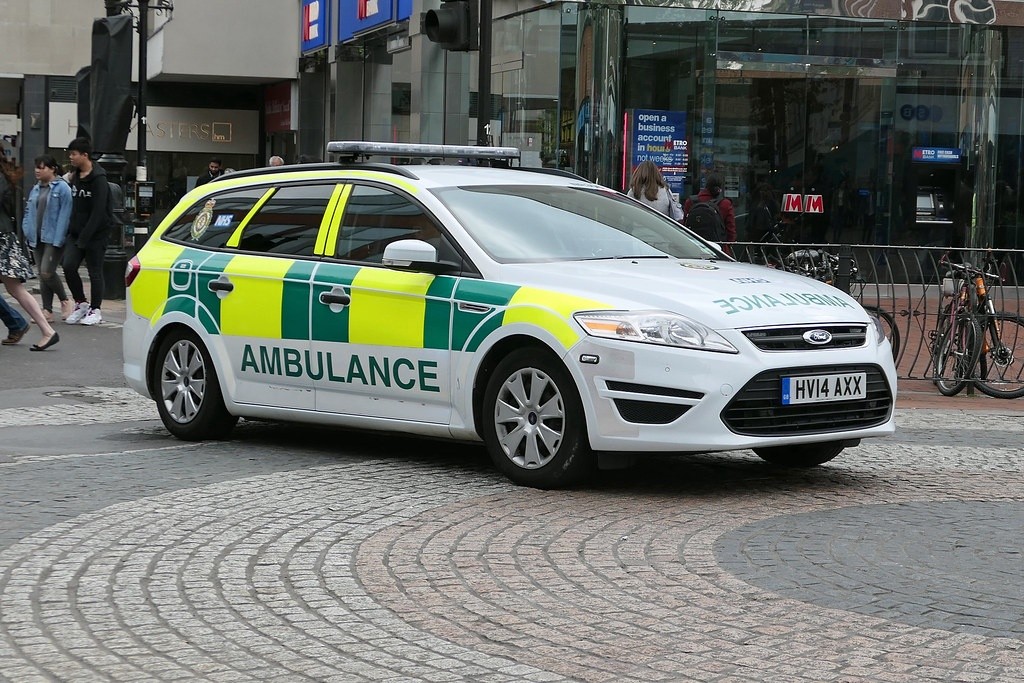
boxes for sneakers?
[60,298,75,320]
[32,309,54,324]
[66,300,102,326]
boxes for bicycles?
[783,248,900,363]
[931,251,1024,397]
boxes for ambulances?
[125,139,898,487]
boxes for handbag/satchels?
[669,188,685,223]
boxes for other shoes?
[30,334,58,352]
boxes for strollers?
[738,243,795,274]
[758,207,806,244]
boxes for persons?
[59,137,112,326]
[683,174,736,258]
[269,156,285,167]
[196,157,224,189]
[0,141,60,350]
[20,154,72,324]
[627,160,675,255]
[0,294,30,344]
[62,164,77,183]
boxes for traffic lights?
[424,0,480,53]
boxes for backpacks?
[687,194,726,245]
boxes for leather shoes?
[2,322,28,345]
